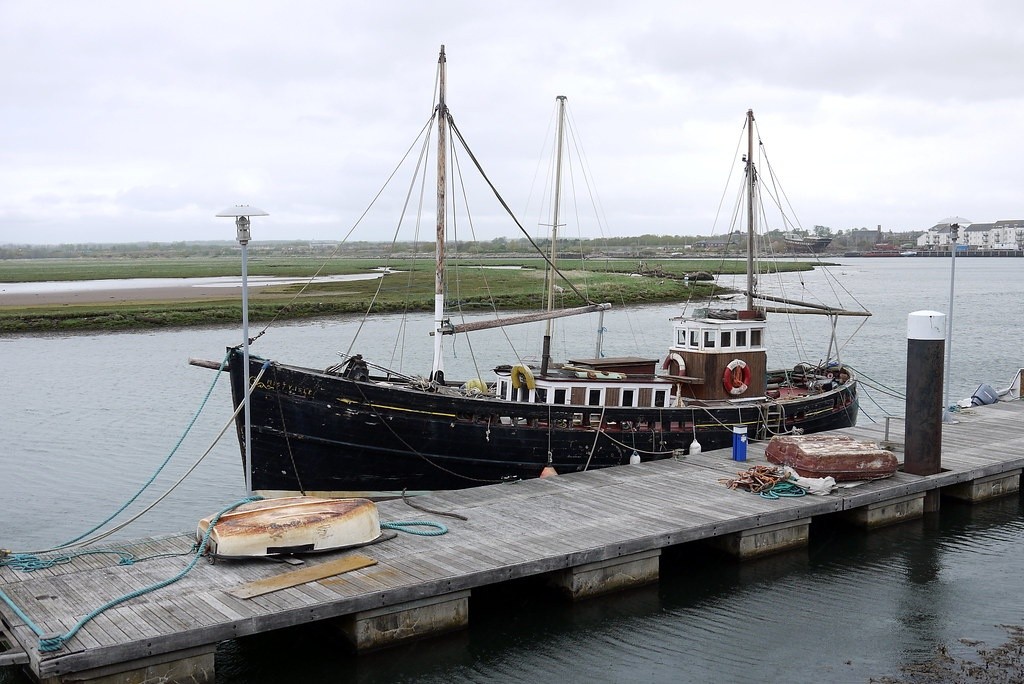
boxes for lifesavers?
[663,352,687,377]
[723,359,751,396]
[511,363,535,389]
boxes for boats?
[213,46,864,496]
